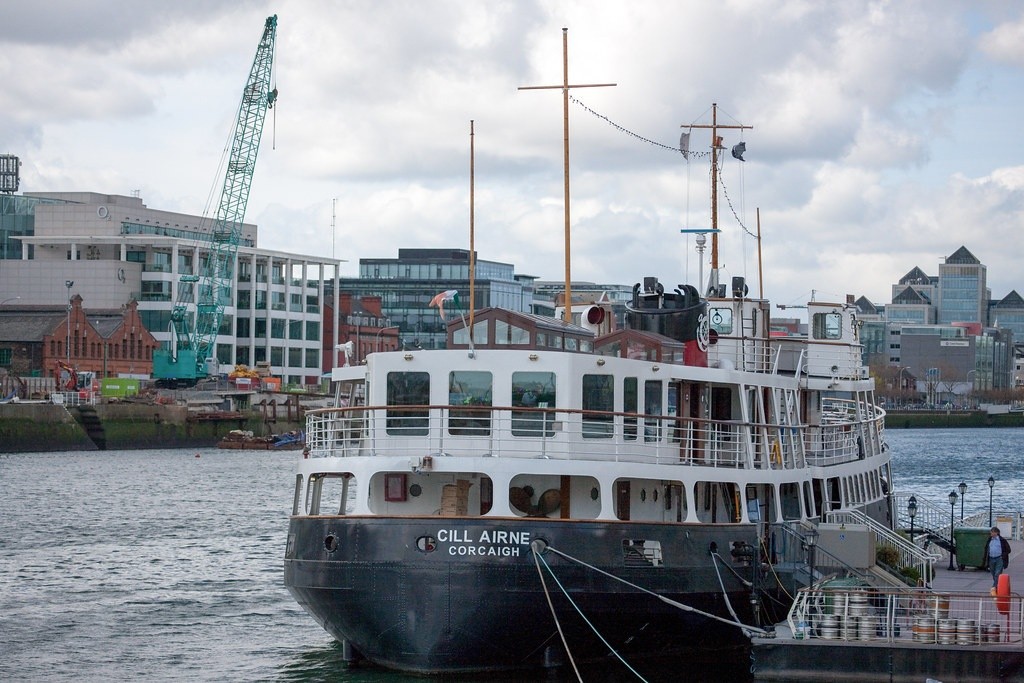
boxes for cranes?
[777,289,846,309]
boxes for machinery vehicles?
[147,14,278,389]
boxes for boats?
[284,26,898,680]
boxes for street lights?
[900,366,911,408]
[928,368,939,408]
[375,324,400,352]
[966,369,976,410]
[353,309,363,367]
[65,280,73,369]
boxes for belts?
[989,555,1002,559]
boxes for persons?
[881,402,980,411]
[984,527,1011,600]
